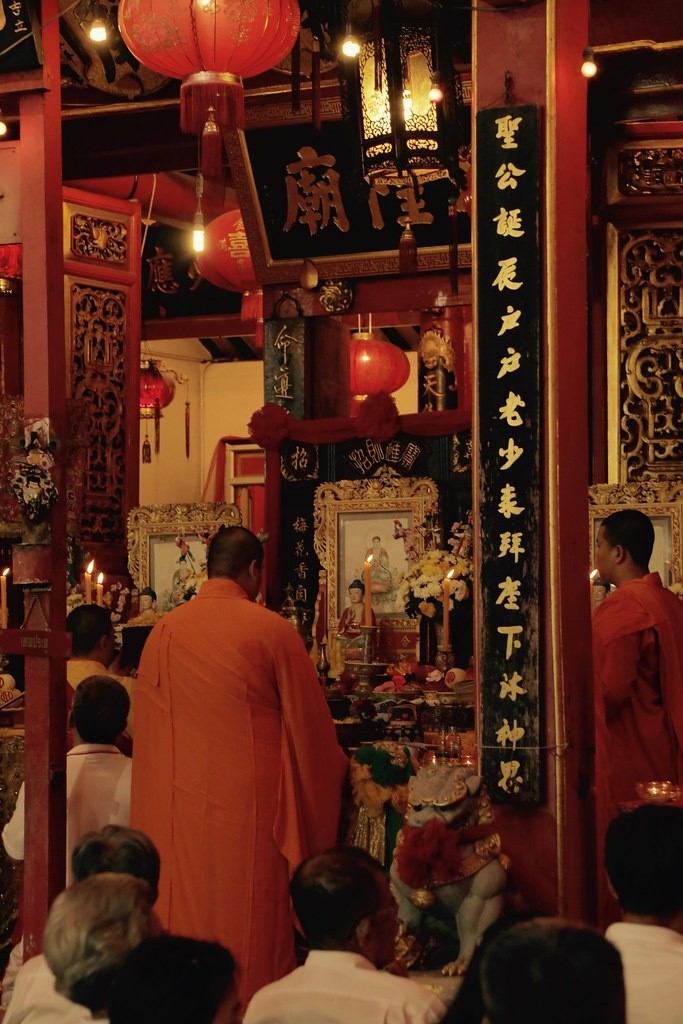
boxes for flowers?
[66,537,130,623]
[393,506,474,621]
[174,521,226,601]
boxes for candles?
[0,576,8,629]
[96,584,104,606]
[442,578,450,647]
[84,572,92,605]
[365,562,372,627]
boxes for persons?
[439,918,627,1024]
[1,824,245,1024]
[240,843,447,1024]
[338,579,377,661]
[592,578,611,610]
[603,800,683,1024]
[137,586,157,615]
[128,526,352,1016]
[64,603,138,758]
[591,508,683,936]
[1,674,133,888]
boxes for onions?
[332,672,360,696]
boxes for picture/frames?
[315,478,441,648]
[589,484,682,598]
[127,503,241,616]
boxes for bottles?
[433,726,461,767]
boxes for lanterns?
[139,359,176,464]
[348,332,411,417]
[117,0,301,178]
[195,208,264,348]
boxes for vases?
[420,615,437,667]
